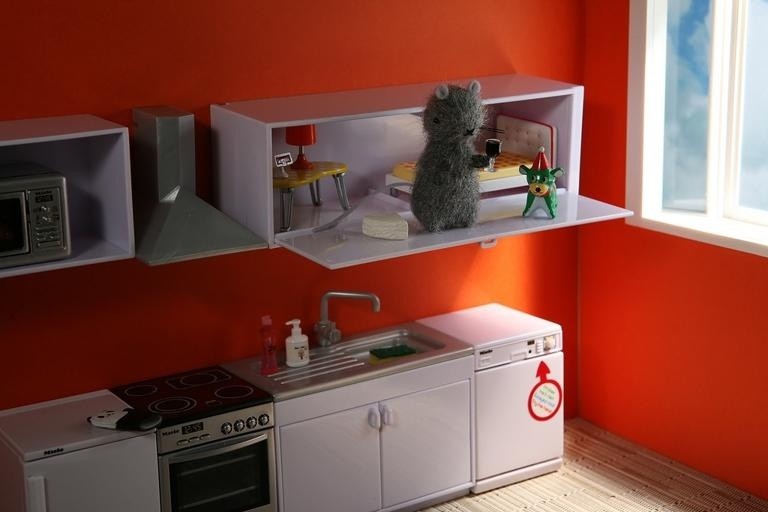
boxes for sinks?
[343,320,475,377]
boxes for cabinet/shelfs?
[207,73,636,273]
[276,355,473,512]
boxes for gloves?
[87,407,163,432]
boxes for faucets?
[314,289,382,346]
